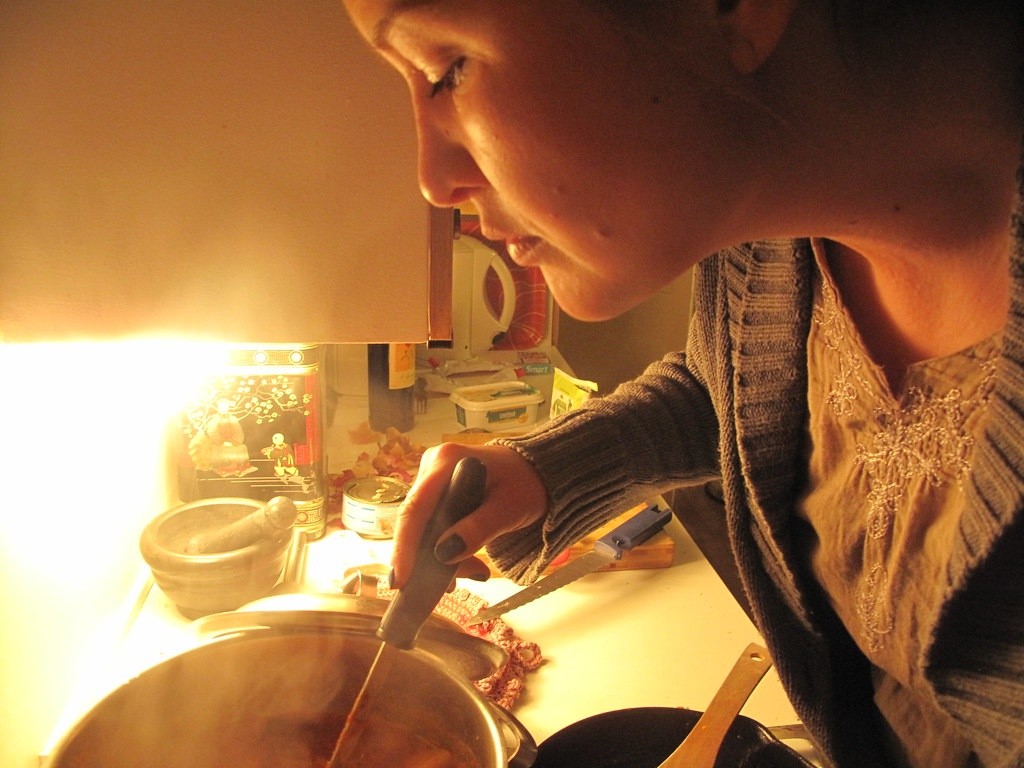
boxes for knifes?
[464,503,672,625]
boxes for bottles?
[367,343,418,432]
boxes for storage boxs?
[448,380,546,430]
[517,351,553,375]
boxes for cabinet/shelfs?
[0,0,454,344]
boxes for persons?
[345,0,1024,768]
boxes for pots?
[526,707,813,768]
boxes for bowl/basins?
[448,380,545,432]
[139,496,297,619]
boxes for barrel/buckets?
[42,625,510,767]
